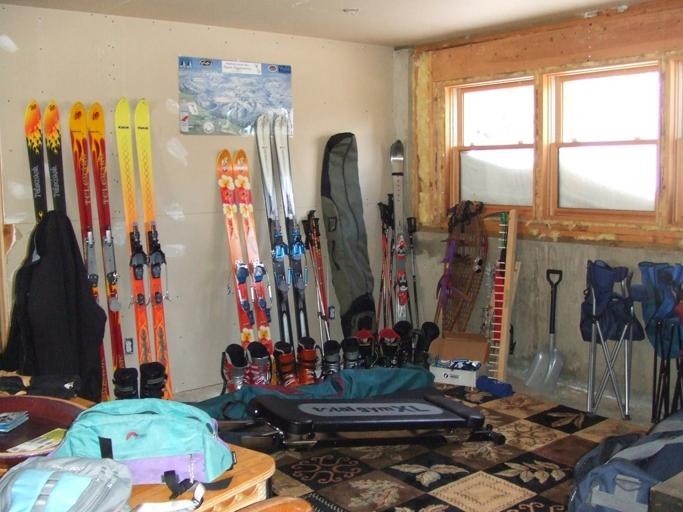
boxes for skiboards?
[68,100,126,402]
[256,114,309,344]
[24,99,66,225]
[216,148,280,387]
[114,97,173,399]
[390,140,408,324]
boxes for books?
[0,411,29,433]
[6,427,68,454]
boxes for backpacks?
[1,454,132,511]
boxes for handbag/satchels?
[47,397,237,485]
[567,407,683,511]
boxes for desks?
[0,392,275,511]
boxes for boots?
[112,362,168,399]
[220,319,440,392]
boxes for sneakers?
[435,355,482,370]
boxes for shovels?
[524,269,566,393]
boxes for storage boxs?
[437,331,490,363]
